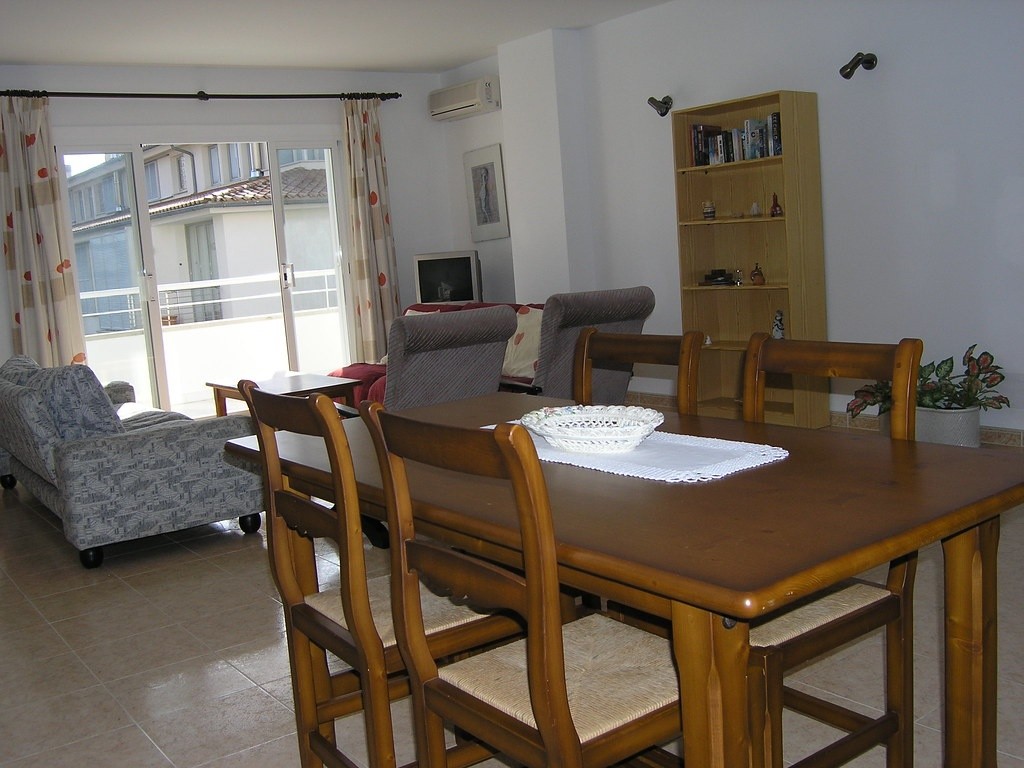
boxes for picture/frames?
[464,144,511,243]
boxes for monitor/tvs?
[413,250,483,304]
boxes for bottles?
[702,201,715,221]
[734,269,744,287]
[770,192,783,217]
[750,200,762,218]
[750,262,765,286]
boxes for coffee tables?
[206,371,363,417]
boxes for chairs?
[360,400,683,768]
[237,380,576,768]
[743,333,923,768]
[573,328,703,415]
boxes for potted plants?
[847,344,1010,448]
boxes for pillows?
[502,305,543,378]
[0,354,126,440]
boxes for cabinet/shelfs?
[671,91,830,429]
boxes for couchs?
[0,376,266,568]
[402,286,656,405]
[328,305,518,421]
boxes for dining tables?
[222,391,1024,768]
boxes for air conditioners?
[427,74,501,123]
